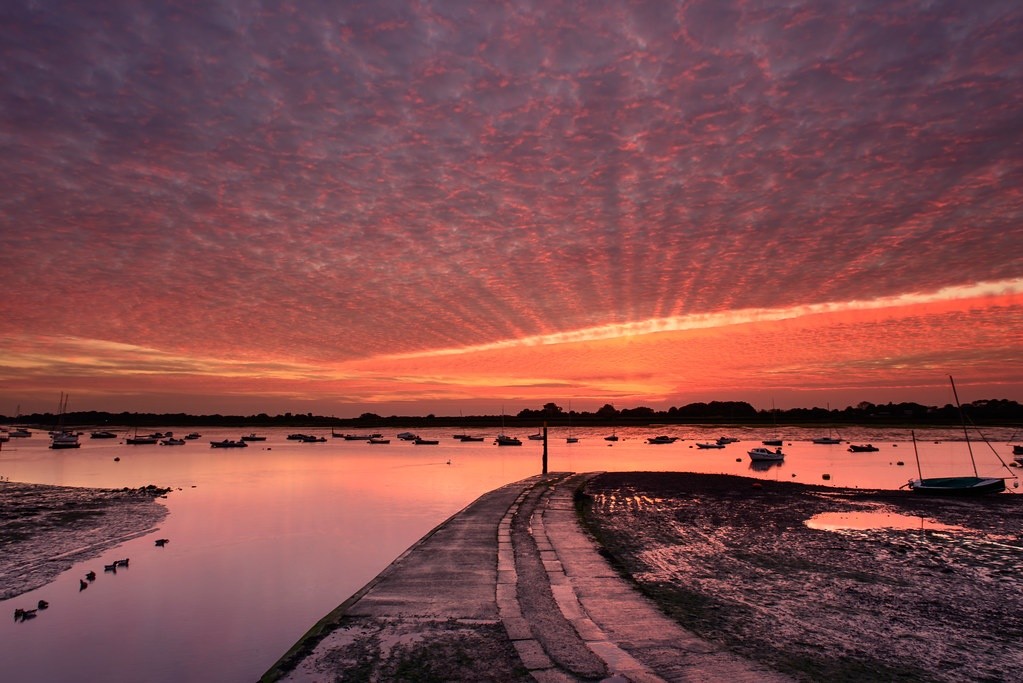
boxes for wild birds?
[14,608,23,616]
[105,561,119,569]
[155,539,169,544]
[21,609,37,617]
[79,579,87,591]
[118,558,130,565]
[38,599,49,606]
[86,570,95,577]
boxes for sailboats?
[813,402,843,444]
[565,400,579,442]
[0,404,32,442]
[48,391,83,448]
[452,409,485,442]
[907,374,1006,495]
[121,412,158,445]
[494,405,523,445]
[329,415,344,437]
[603,425,619,441]
[761,397,783,446]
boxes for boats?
[90,431,117,438]
[528,425,544,440]
[746,447,785,461]
[396,431,440,445]
[849,443,880,452]
[343,432,390,445]
[209,433,267,447]
[648,436,679,444]
[150,431,202,445]
[286,433,328,443]
[696,437,741,449]
